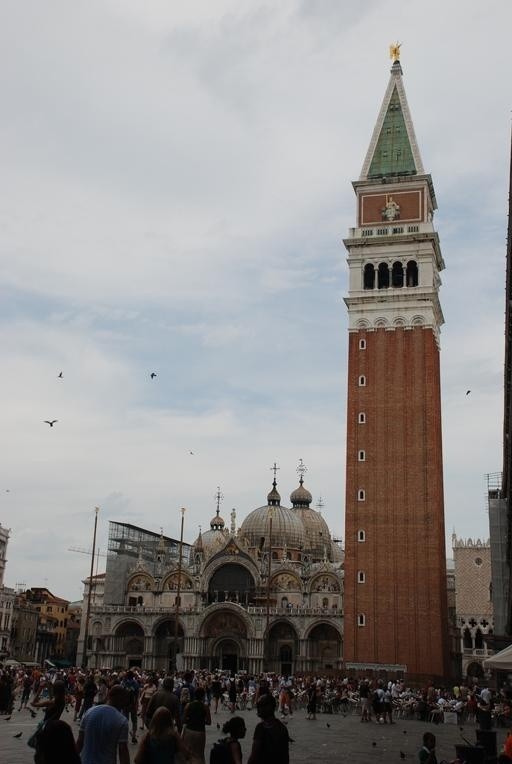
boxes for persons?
[0,663,512,764]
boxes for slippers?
[210,736,239,763]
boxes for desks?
[172,507,186,676]
[81,506,101,671]
[262,506,276,659]
